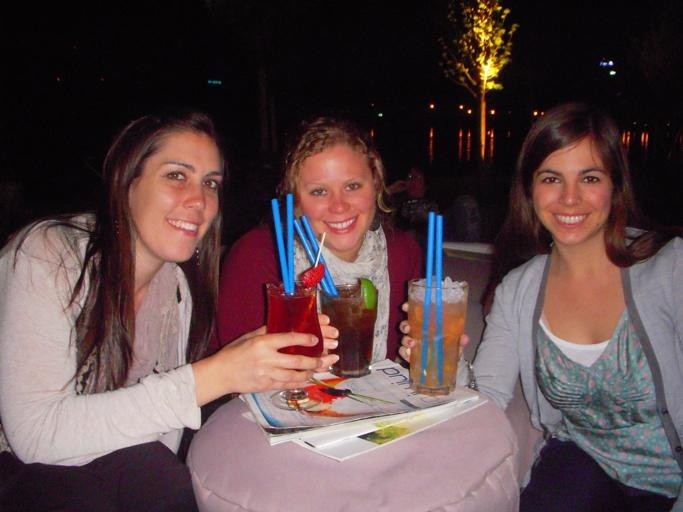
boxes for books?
[234,358,453,444]
[240,383,477,450]
[240,394,492,462]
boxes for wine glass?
[263,281,324,407]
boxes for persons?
[377,188,421,355]
[0,106,338,510]
[207,114,424,396]
[439,192,502,244]
[382,161,441,230]
[397,99,683,511]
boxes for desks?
[443,218,650,487]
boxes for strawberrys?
[301,264,324,289]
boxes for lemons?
[359,279,377,310]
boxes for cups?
[320,284,380,376]
[404,276,470,393]
